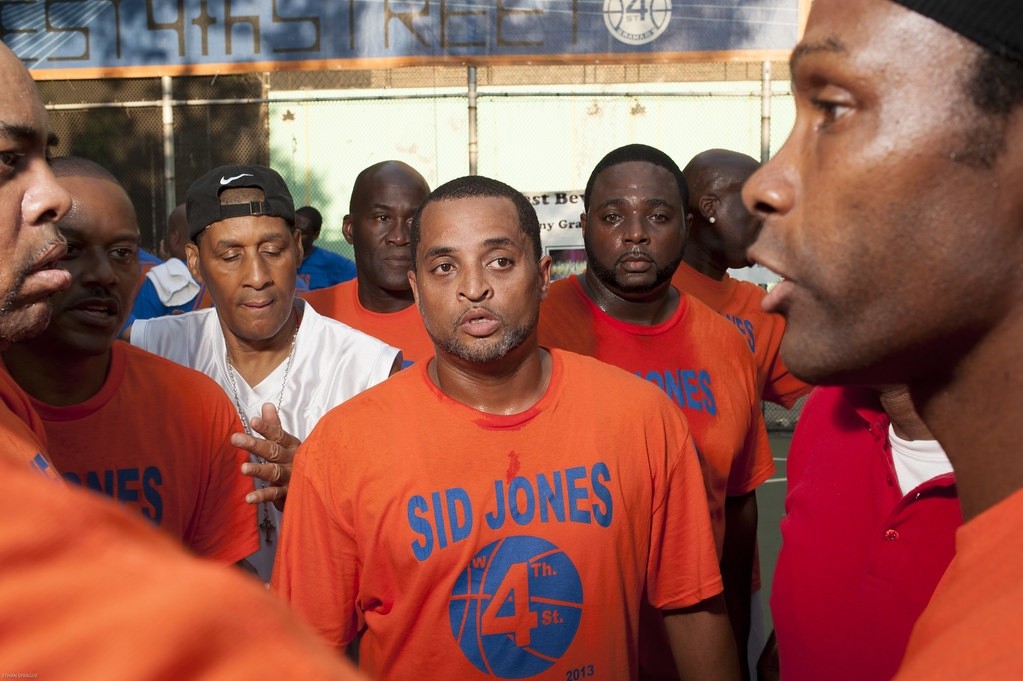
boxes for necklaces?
[218,303,299,548]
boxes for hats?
[186,166,295,240]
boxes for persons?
[0,36,365,679]
[296,157,437,374]
[529,144,776,680]
[756,354,964,679]
[288,205,359,288]
[3,146,262,581]
[671,149,818,681]
[266,174,745,680]
[734,1,1020,680]
[133,201,210,320]
[115,164,404,584]
